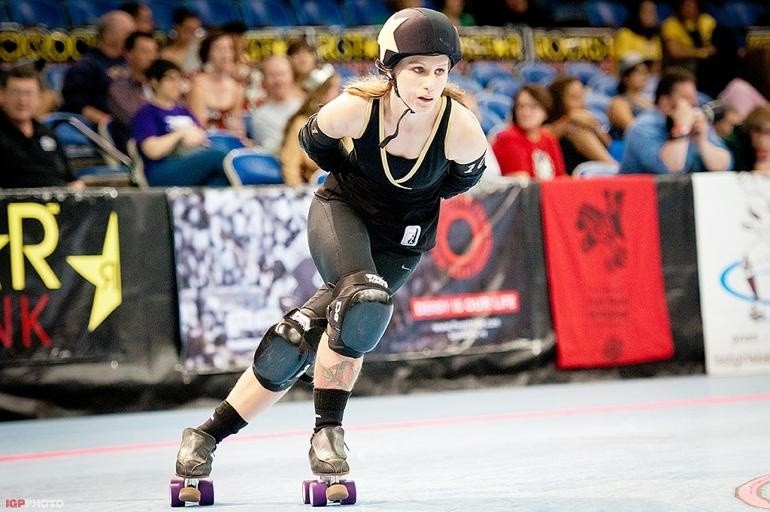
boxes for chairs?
[1,58,735,188]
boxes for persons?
[173,6,489,478]
[2,1,770,187]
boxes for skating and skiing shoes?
[302,426,356,507]
[169,427,216,507]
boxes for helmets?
[373,7,462,74]
